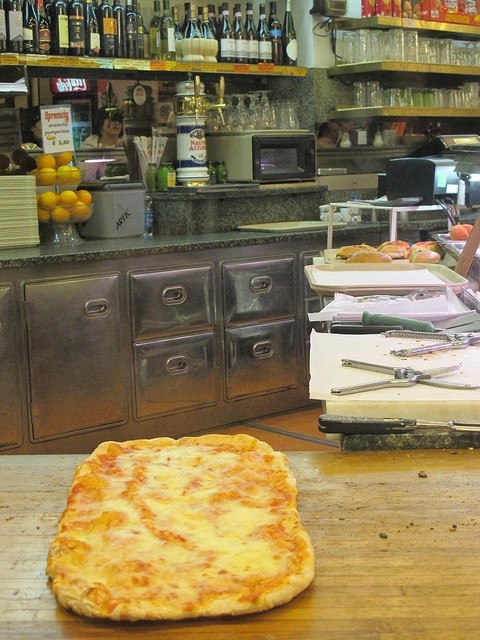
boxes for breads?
[451,225,467,241]
[337,240,443,265]
[462,224,473,241]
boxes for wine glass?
[221,95,235,128]
[204,94,223,131]
[247,93,264,131]
[231,93,249,131]
[256,90,275,129]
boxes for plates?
[0,175,40,249]
[26,169,94,243]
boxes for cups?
[433,39,452,65]
[367,30,382,64]
[353,29,370,63]
[383,87,399,107]
[366,80,382,106]
[426,88,440,108]
[453,46,480,66]
[457,89,465,111]
[439,88,447,109]
[411,90,424,108]
[422,91,433,108]
[418,40,433,64]
[398,87,413,107]
[276,100,301,131]
[386,29,404,62]
[341,32,355,65]
[353,81,365,106]
[446,90,458,110]
[404,31,418,62]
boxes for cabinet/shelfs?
[3,48,306,81]
[335,14,478,121]
[126,235,300,441]
[24,274,133,444]
[0,282,22,452]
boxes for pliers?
[331,359,479,398]
[386,329,480,357]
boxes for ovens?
[205,131,316,184]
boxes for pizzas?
[46,434,314,624]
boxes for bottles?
[207,160,217,185]
[112,0,126,57]
[268,1,284,65]
[22,0,40,54]
[0,0,8,53]
[244,3,259,64]
[233,4,248,64]
[217,2,237,64]
[216,160,229,185]
[7,0,25,53]
[149,0,162,59]
[67,0,86,56]
[146,161,162,192]
[180,2,191,39]
[282,0,298,66]
[217,6,222,30]
[51,0,70,55]
[172,6,181,41]
[132,0,144,59]
[208,4,218,35]
[166,162,177,190]
[96,0,115,58]
[200,7,216,64]
[85,0,102,57]
[125,0,139,58]
[158,161,170,191]
[256,4,273,64]
[184,4,201,59]
[35,0,52,55]
[160,0,176,61]
[141,194,154,238]
